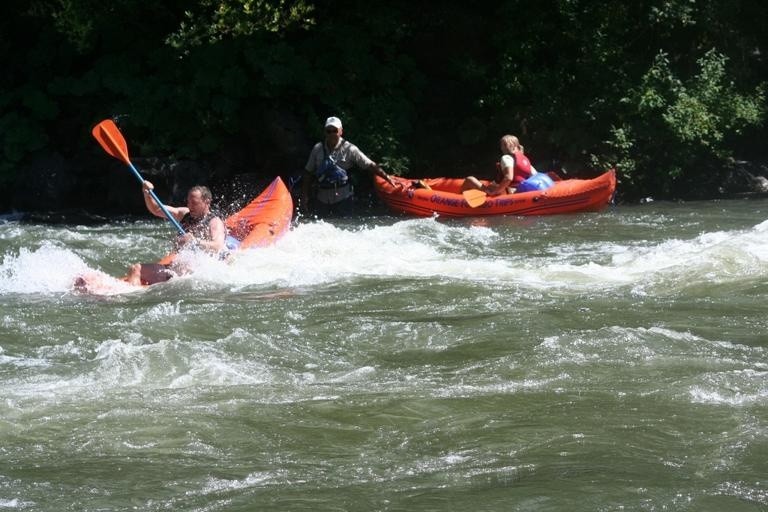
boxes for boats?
[372,167,617,219]
[74,174,294,294]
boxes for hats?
[325,116,343,129]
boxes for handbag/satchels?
[313,152,348,188]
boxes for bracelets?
[385,174,390,181]
[196,238,201,246]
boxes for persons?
[73,178,228,300]
[300,116,406,218]
[456,134,537,199]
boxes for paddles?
[463,189,487,209]
[92,120,186,236]
